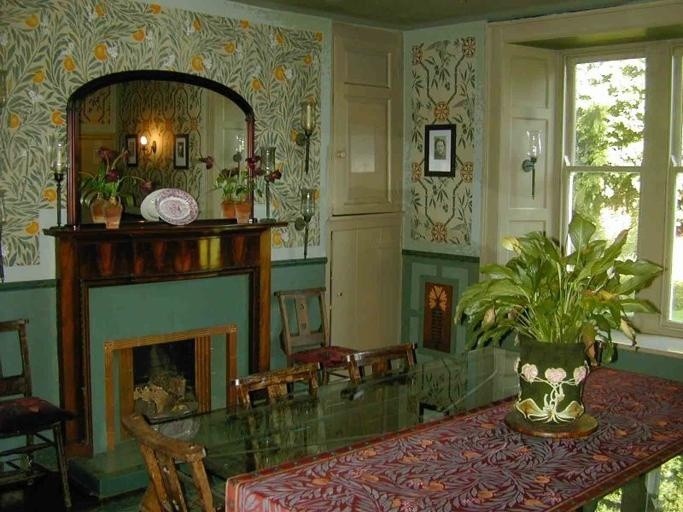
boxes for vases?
[234,191,258,225]
[90,192,106,224]
[507,336,591,425]
[220,198,235,218]
[102,198,123,229]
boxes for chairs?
[231,361,321,410]
[274,288,357,385]
[0,318,76,512]
[343,342,417,384]
[122,414,221,512]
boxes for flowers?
[80,145,132,205]
[237,155,282,194]
[199,152,248,199]
[91,149,152,202]
[455,211,667,367]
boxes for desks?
[148,347,683,511]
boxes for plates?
[156,189,199,225]
[140,187,169,223]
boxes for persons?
[434,138,447,160]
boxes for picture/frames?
[125,134,137,167]
[425,124,456,177]
[174,134,189,168]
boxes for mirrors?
[65,70,255,224]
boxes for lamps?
[260,146,278,225]
[522,128,542,197]
[140,135,156,154]
[296,100,316,174]
[295,188,314,259]
